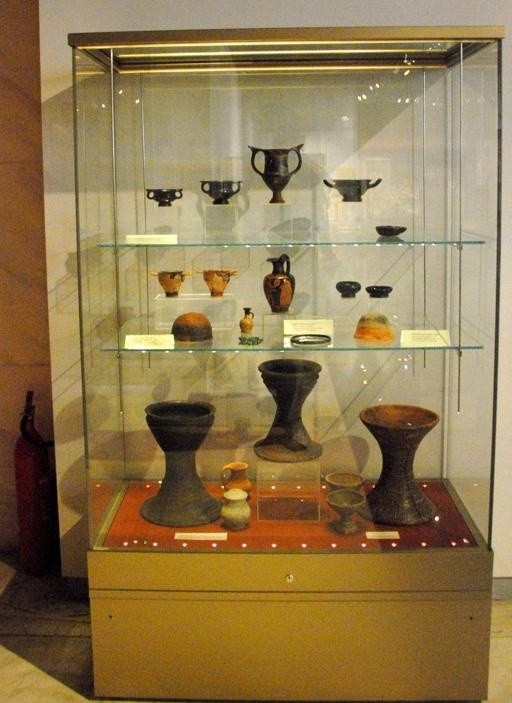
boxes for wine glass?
[324,473,366,535]
[358,405,438,525]
[253,358,324,461]
[145,400,219,525]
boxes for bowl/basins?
[337,281,361,298]
[366,286,392,298]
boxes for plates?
[376,225,407,237]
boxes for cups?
[146,188,183,206]
[201,181,240,204]
[249,144,303,204]
[151,270,190,298]
[194,269,237,298]
[324,178,381,202]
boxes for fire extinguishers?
[14,390,55,578]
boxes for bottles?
[240,307,255,339]
[220,462,252,530]
[263,253,296,313]
